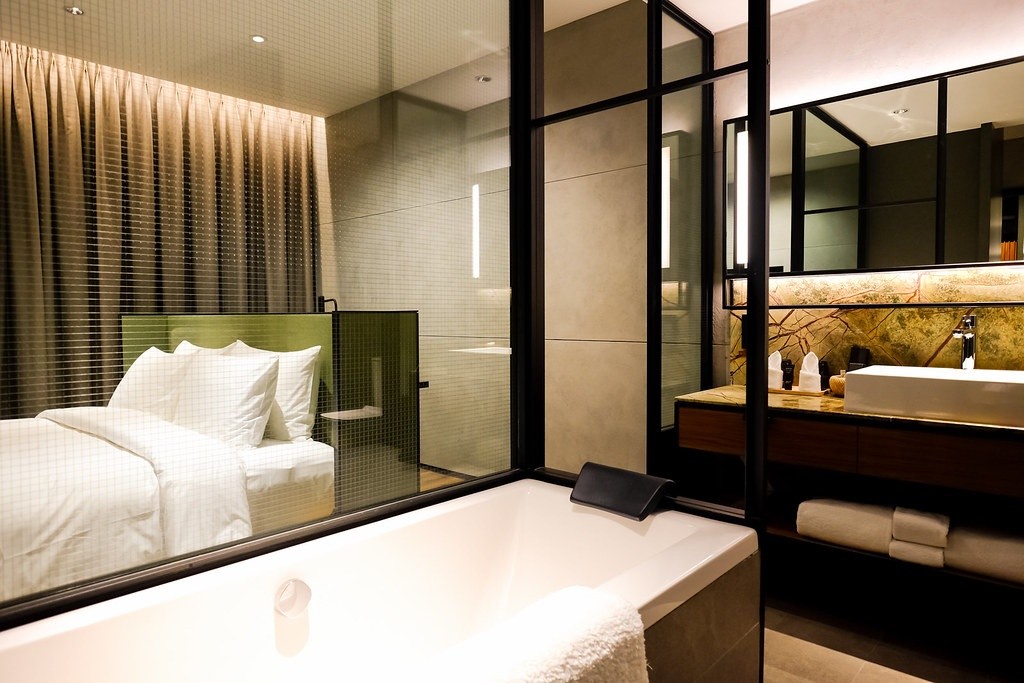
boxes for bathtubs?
[0,478,765,683]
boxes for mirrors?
[720,55,1024,268]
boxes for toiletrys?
[780,357,796,389]
[818,360,831,380]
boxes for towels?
[797,351,822,394]
[796,498,1024,581]
[517,602,651,683]
[768,350,783,390]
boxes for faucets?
[951,314,978,369]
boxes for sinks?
[842,364,1024,428]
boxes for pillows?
[172,355,280,447]
[106,349,186,420]
[236,338,322,444]
[172,340,236,358]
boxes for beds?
[0,417,336,603]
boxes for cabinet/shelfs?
[765,519,1024,679]
[672,403,1024,493]
